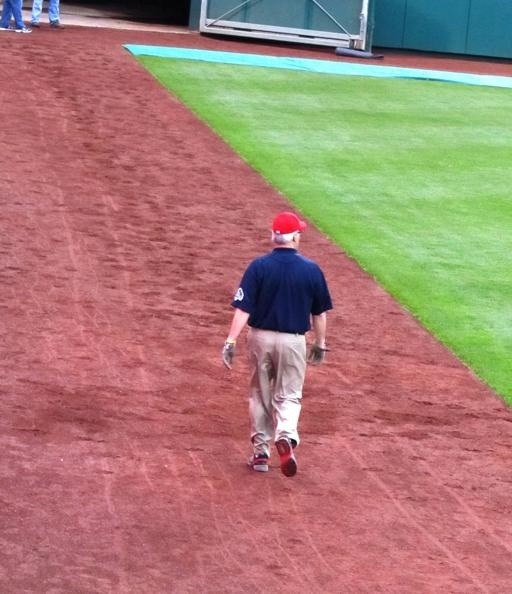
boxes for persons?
[28,0,64,28]
[0,0,31,33]
[221,212,332,476]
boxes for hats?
[273,212,306,234]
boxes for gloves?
[222,341,235,370]
[307,341,329,365]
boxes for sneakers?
[248,452,269,471]
[274,438,297,476]
[0,22,64,33]
